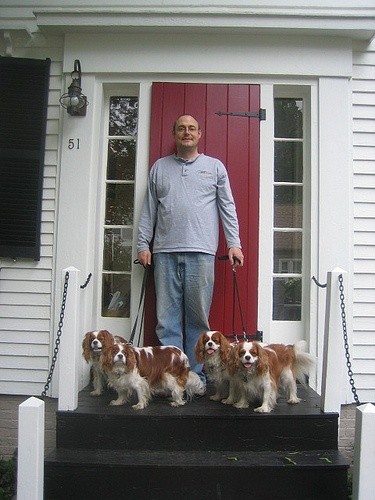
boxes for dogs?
[195,330,321,413]
[81,330,206,410]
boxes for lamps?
[59,59,87,116]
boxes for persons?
[136,114,244,386]
